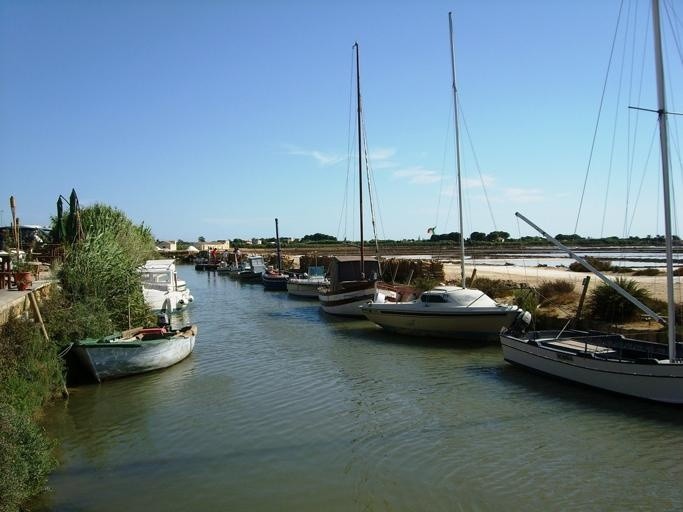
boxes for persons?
[212,248,241,264]
[158,309,169,328]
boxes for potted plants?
[7,245,37,290]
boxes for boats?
[195,250,332,296]
[65,322,200,385]
[129,259,194,314]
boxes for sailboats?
[494,1,683,412]
[356,10,532,337]
[318,41,400,317]
